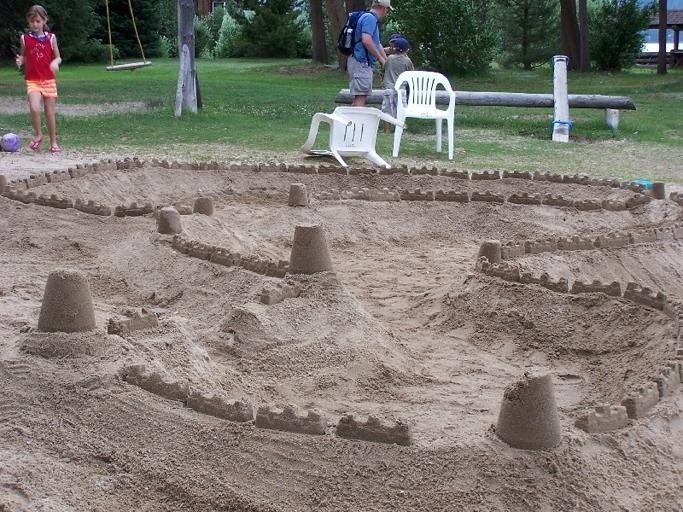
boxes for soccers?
[2,133,20,151]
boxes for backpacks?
[338,9,378,55]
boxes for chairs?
[391,69,457,161]
[299,105,404,169]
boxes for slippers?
[28,136,42,149]
[51,144,58,151]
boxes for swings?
[105,1,152,72]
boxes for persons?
[347,0,394,106]
[15,4,63,152]
[382,34,401,81]
[381,38,415,134]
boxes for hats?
[372,0,394,11]
[390,39,408,49]
